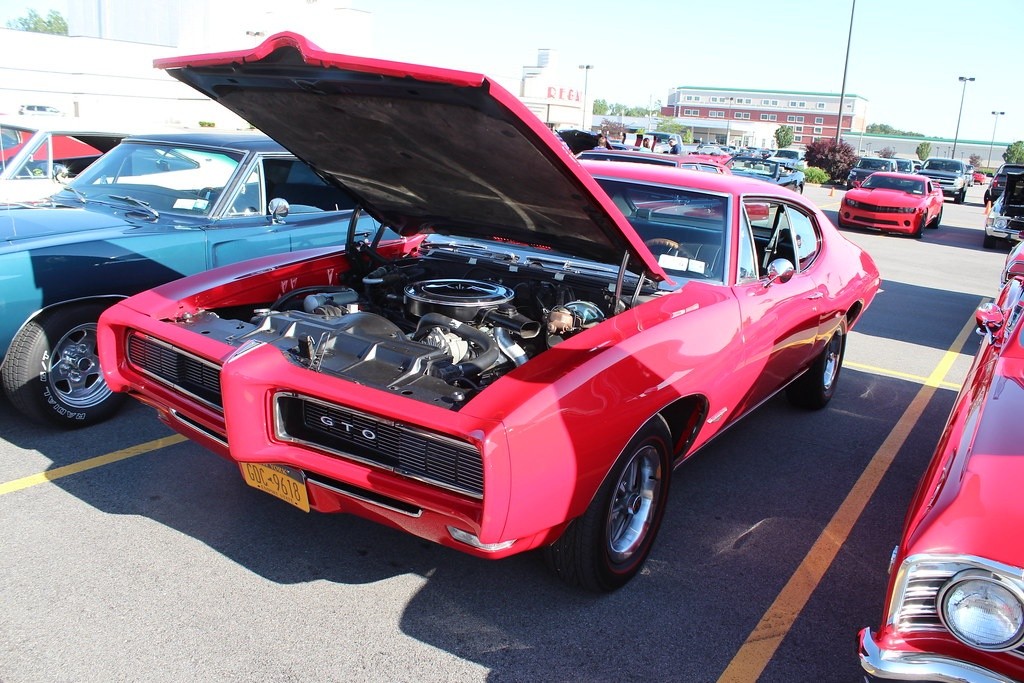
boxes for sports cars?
[723,155,806,196]
[838,172,945,240]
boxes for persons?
[594,137,680,156]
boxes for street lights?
[987,111,1005,169]
[951,77,976,158]
[866,142,964,161]
[578,65,593,129]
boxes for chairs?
[664,242,724,282]
[755,162,776,173]
[931,163,943,170]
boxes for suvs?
[847,157,897,190]
[912,159,970,204]
[983,163,1024,203]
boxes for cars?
[0,105,262,207]
[91,32,886,592]
[891,157,925,185]
[0,132,403,432]
[555,126,807,224]
[858,237,1024,681]
[983,171,1024,248]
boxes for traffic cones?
[984,200,992,215]
[829,187,836,197]
